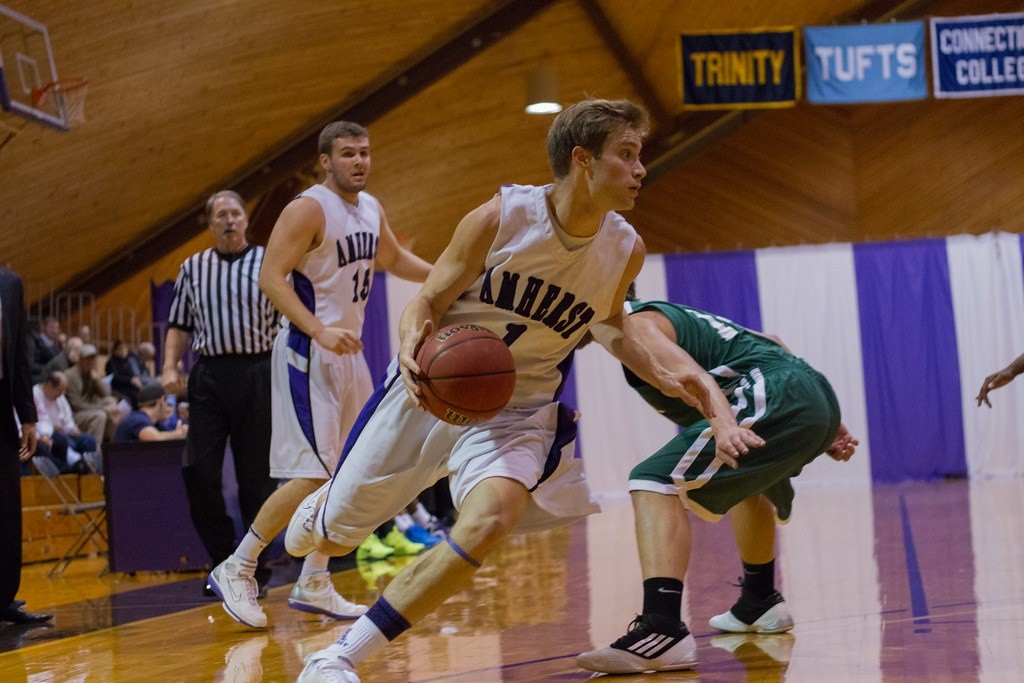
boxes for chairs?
[34,453,111,579]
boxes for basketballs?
[411,323,517,428]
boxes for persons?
[13,317,190,480]
[284,100,717,683]
[111,382,189,444]
[976,354,1024,407]
[208,122,435,628]
[160,192,293,600]
[0,270,53,623]
[571,280,859,674]
[355,498,451,562]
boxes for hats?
[137,382,163,405]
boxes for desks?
[101,443,290,576]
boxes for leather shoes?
[0,608,53,623]
[0,621,29,636]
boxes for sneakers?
[576,615,699,673]
[405,524,442,549]
[288,570,369,620]
[296,650,361,683]
[382,527,426,555]
[710,633,795,675]
[285,478,333,557]
[218,636,268,683]
[708,593,794,633]
[426,516,451,537]
[208,555,268,627]
[291,621,356,667]
[356,534,396,560]
